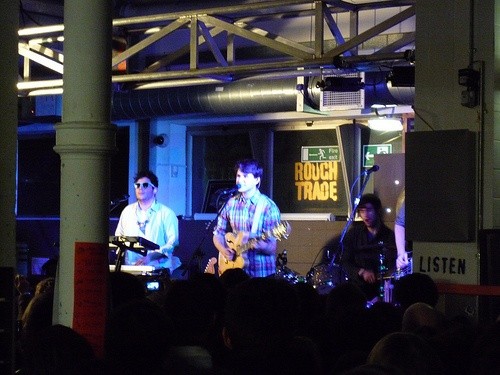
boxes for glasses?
[133,182,156,189]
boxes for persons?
[212,159,280,278]
[14,268,500,375]
[115,170,180,276]
[338,193,398,302]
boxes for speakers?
[405,128,475,243]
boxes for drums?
[306,262,349,292]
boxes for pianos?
[108,265,170,281]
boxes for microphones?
[224,185,242,194]
[111,195,130,204]
[362,166,379,175]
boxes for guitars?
[204,256,216,275]
[217,220,290,276]
